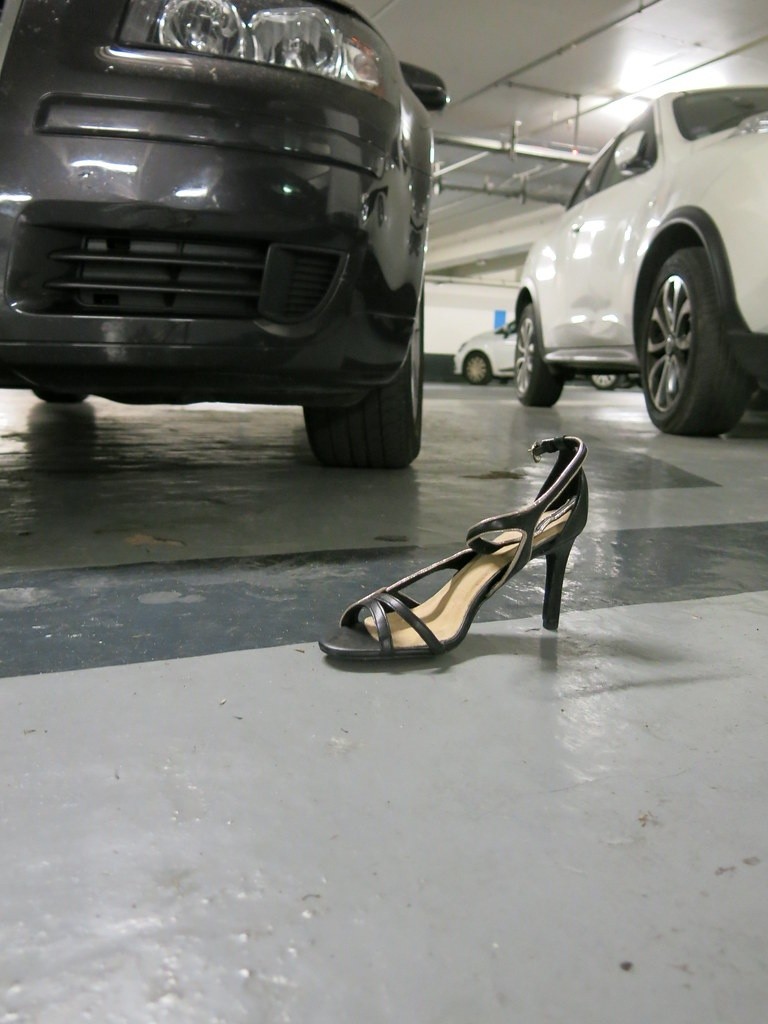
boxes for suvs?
[1,1,451,472]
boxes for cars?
[514,78,767,437]
[453,315,618,393]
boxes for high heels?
[317,436,590,664]
